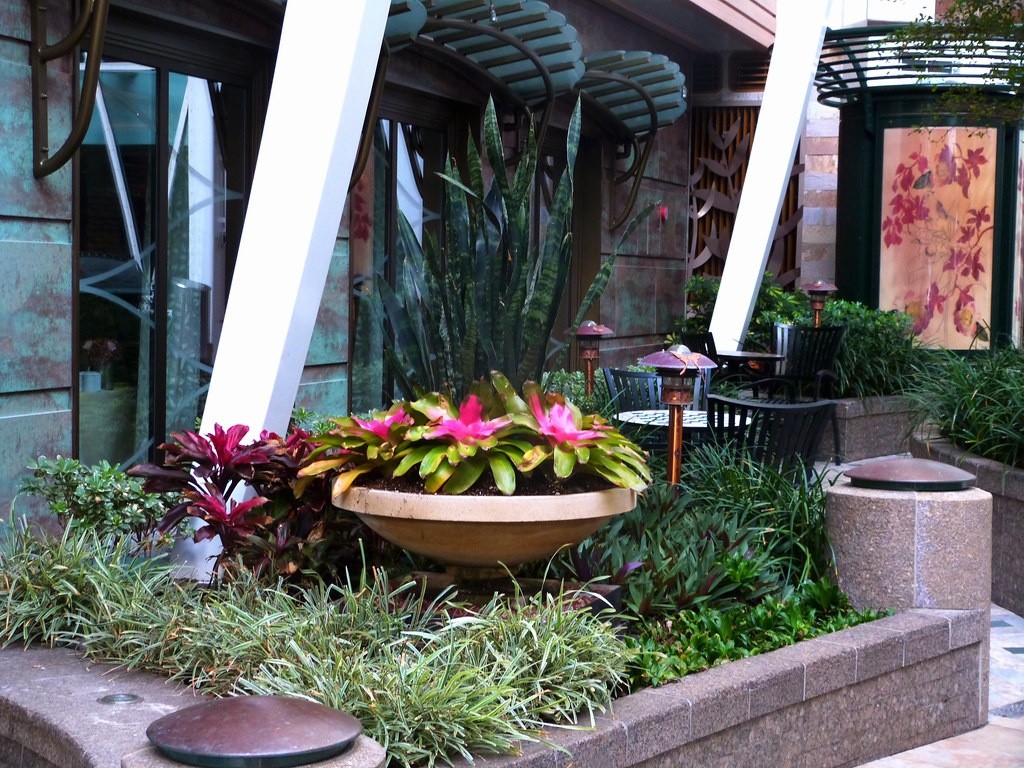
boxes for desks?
[709,350,784,399]
[611,406,754,465]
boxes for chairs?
[742,320,841,466]
[758,323,796,390]
[681,330,720,361]
[602,367,702,450]
[702,391,838,495]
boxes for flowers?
[290,92,658,500]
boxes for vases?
[326,471,640,596]
